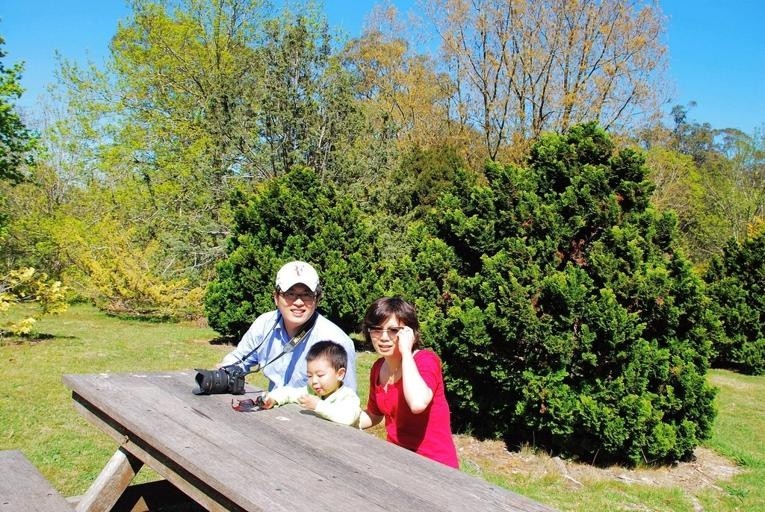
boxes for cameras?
[191,363,247,396]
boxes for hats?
[276,261,319,293]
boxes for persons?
[214,261,358,396]
[254,338,362,430]
[358,296,462,471]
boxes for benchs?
[0,449,76,512]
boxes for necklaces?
[389,358,401,383]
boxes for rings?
[406,328,410,332]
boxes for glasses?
[232,396,263,412]
[366,326,403,339]
[278,290,316,301]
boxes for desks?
[62,369,560,512]
